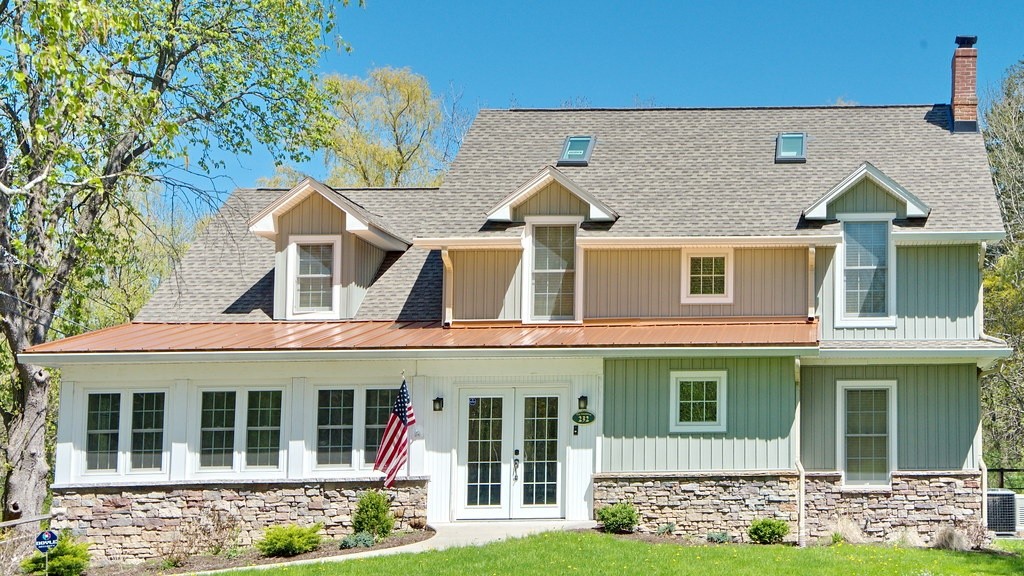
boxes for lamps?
[432,397,443,411]
[578,396,588,409]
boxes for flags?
[373,379,417,489]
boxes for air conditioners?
[987,487,1017,538]
